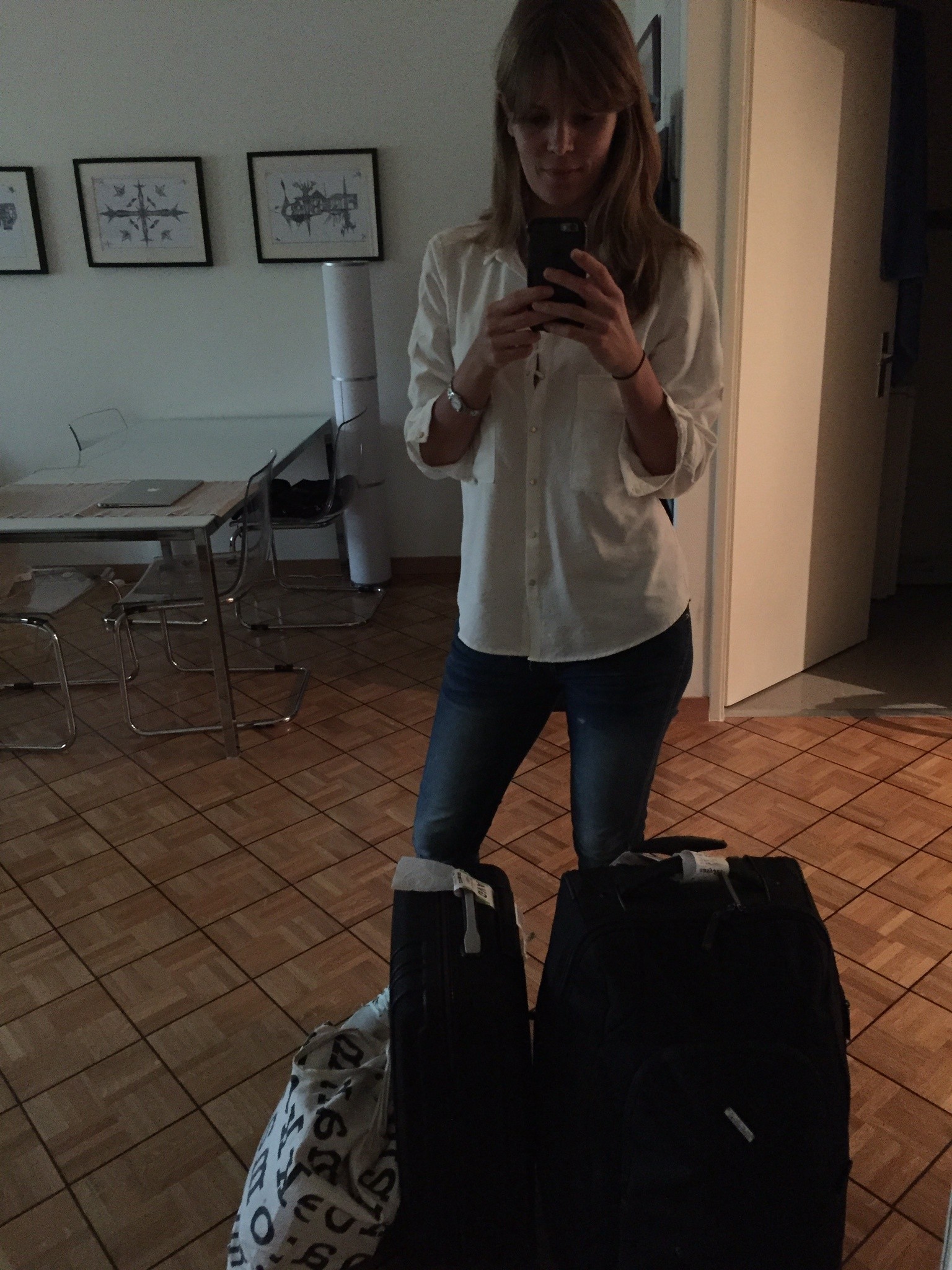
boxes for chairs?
[0,406,387,753]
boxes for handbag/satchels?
[227,1023,399,1270]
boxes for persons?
[334,0,736,1047]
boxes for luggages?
[533,836,853,1270]
[385,858,532,1270]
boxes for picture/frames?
[247,148,384,263]
[0,165,49,275]
[72,156,213,268]
[635,14,660,123]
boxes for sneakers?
[340,987,391,1041]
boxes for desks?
[0,418,349,761]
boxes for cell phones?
[526,216,587,333]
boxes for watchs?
[447,375,490,416]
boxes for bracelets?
[612,350,645,380]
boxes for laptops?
[98,477,203,508]
